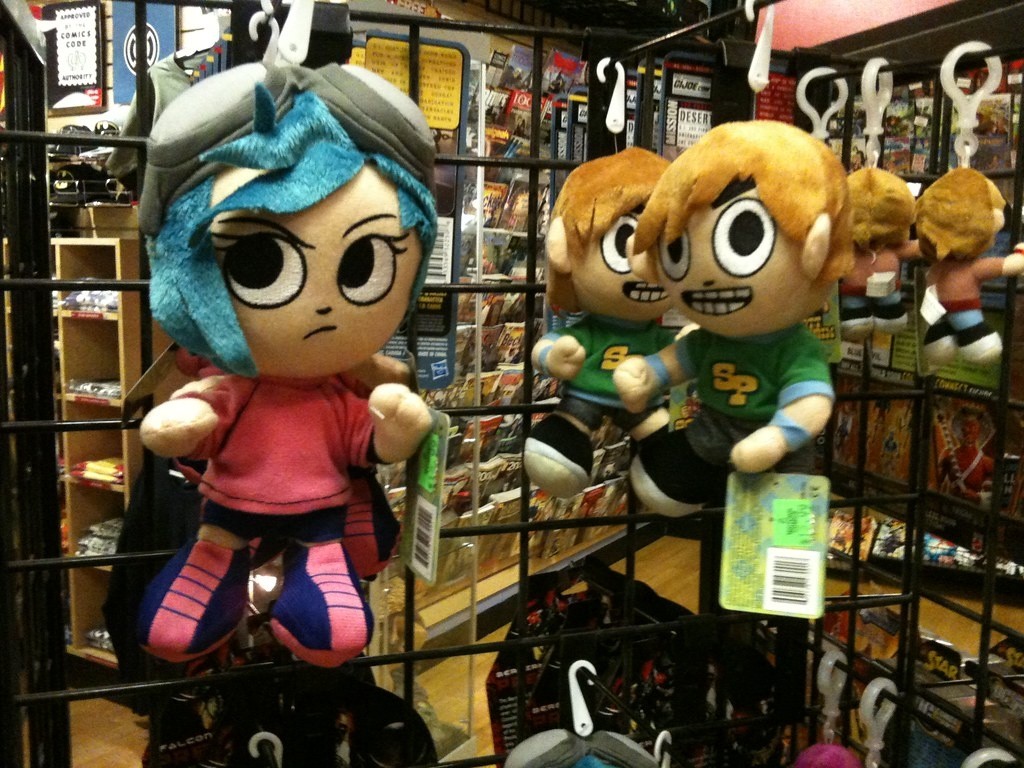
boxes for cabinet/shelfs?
[4,235,145,673]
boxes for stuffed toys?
[839,164,923,339]
[131,59,440,669]
[520,120,854,517]
[911,166,1024,364]
